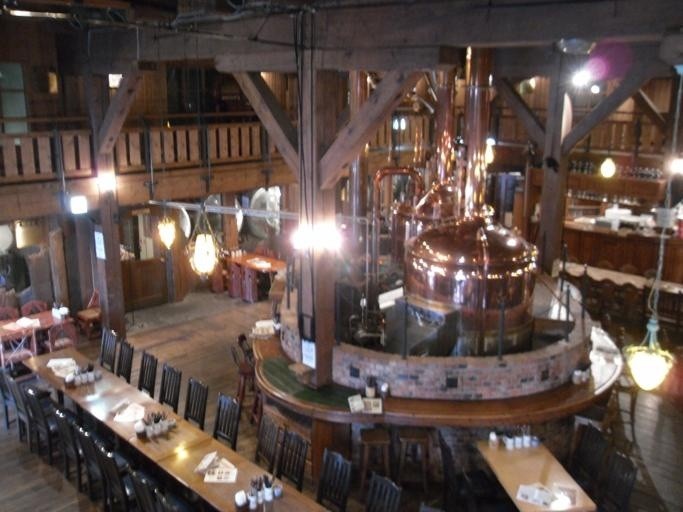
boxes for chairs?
[0,288,102,367]
[558,424,606,489]
[597,454,638,511]
[460,467,514,511]
[212,262,259,302]
[560,251,683,346]
[438,432,494,512]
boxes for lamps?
[67,194,88,216]
[600,113,617,178]
[191,206,218,277]
[156,204,176,251]
[621,73,683,393]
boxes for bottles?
[380,382,389,400]
[365,375,377,399]
[489,431,499,452]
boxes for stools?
[611,372,637,426]
[399,429,430,506]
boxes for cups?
[145,425,155,439]
[264,484,273,502]
[505,437,515,451]
[87,369,96,383]
[273,485,284,497]
[258,489,264,504]
[523,435,531,448]
[74,375,81,387]
[514,436,524,449]
[153,422,162,436]
[80,372,88,385]
[248,495,257,509]
[161,419,169,432]
